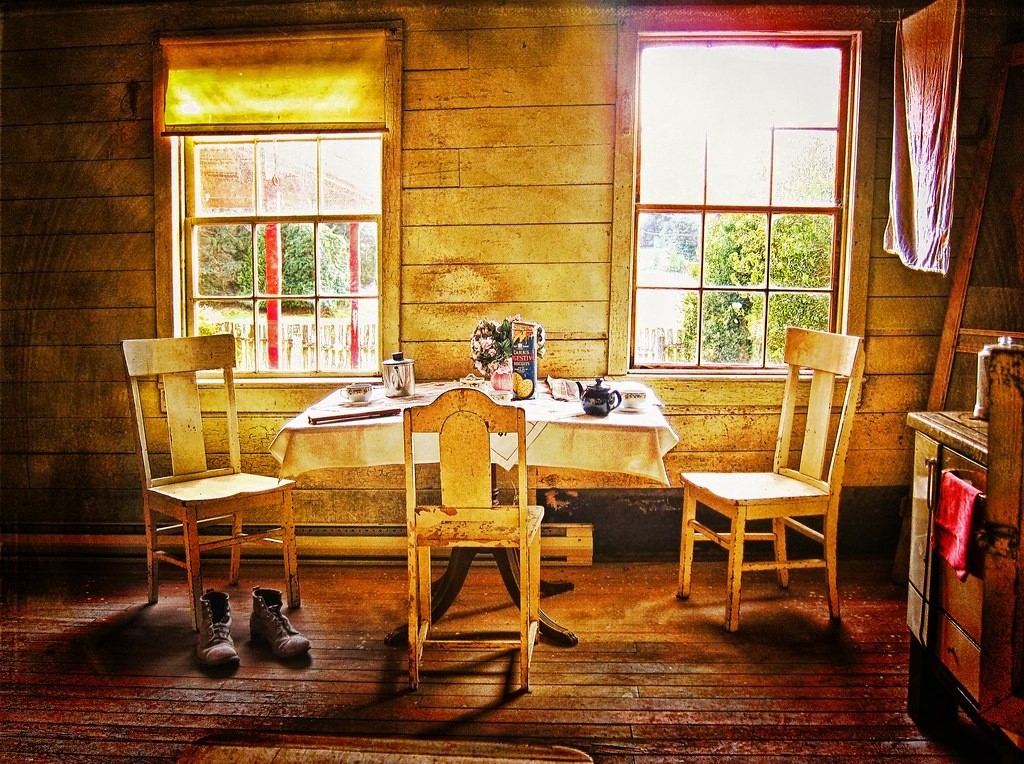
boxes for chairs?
[120,333,301,633]
[403,387,545,690]
[676,326,866,634]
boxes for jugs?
[382,352,416,397]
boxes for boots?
[197,588,240,666]
[250,585,311,657]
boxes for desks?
[900,409,1024,764]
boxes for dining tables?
[284,380,667,646]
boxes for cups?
[973,336,1024,419]
[339,384,372,402]
[618,389,646,412]
[489,390,510,404]
[460,376,484,389]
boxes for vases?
[490,369,514,391]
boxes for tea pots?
[575,377,622,417]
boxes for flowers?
[469,312,547,374]
